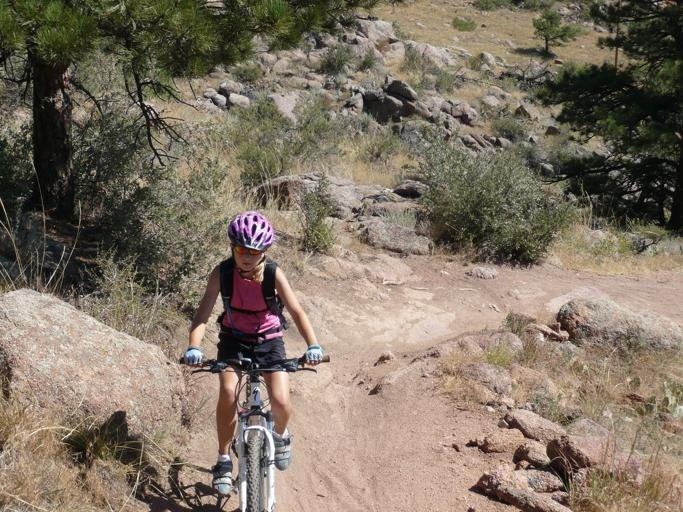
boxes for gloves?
[304,345,325,366]
[184,345,205,367]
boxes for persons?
[180,210,326,499]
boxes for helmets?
[227,210,275,253]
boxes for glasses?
[231,242,265,256]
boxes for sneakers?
[211,459,235,497]
[270,424,294,473]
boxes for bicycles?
[178,351,329,512]
